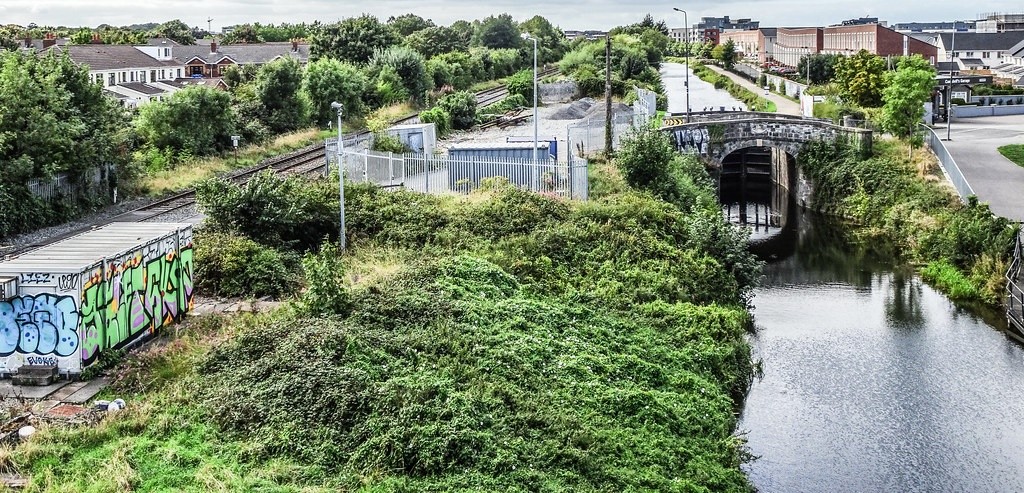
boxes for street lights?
[331,102,346,256]
[520,32,538,192]
[673,7,689,123]
